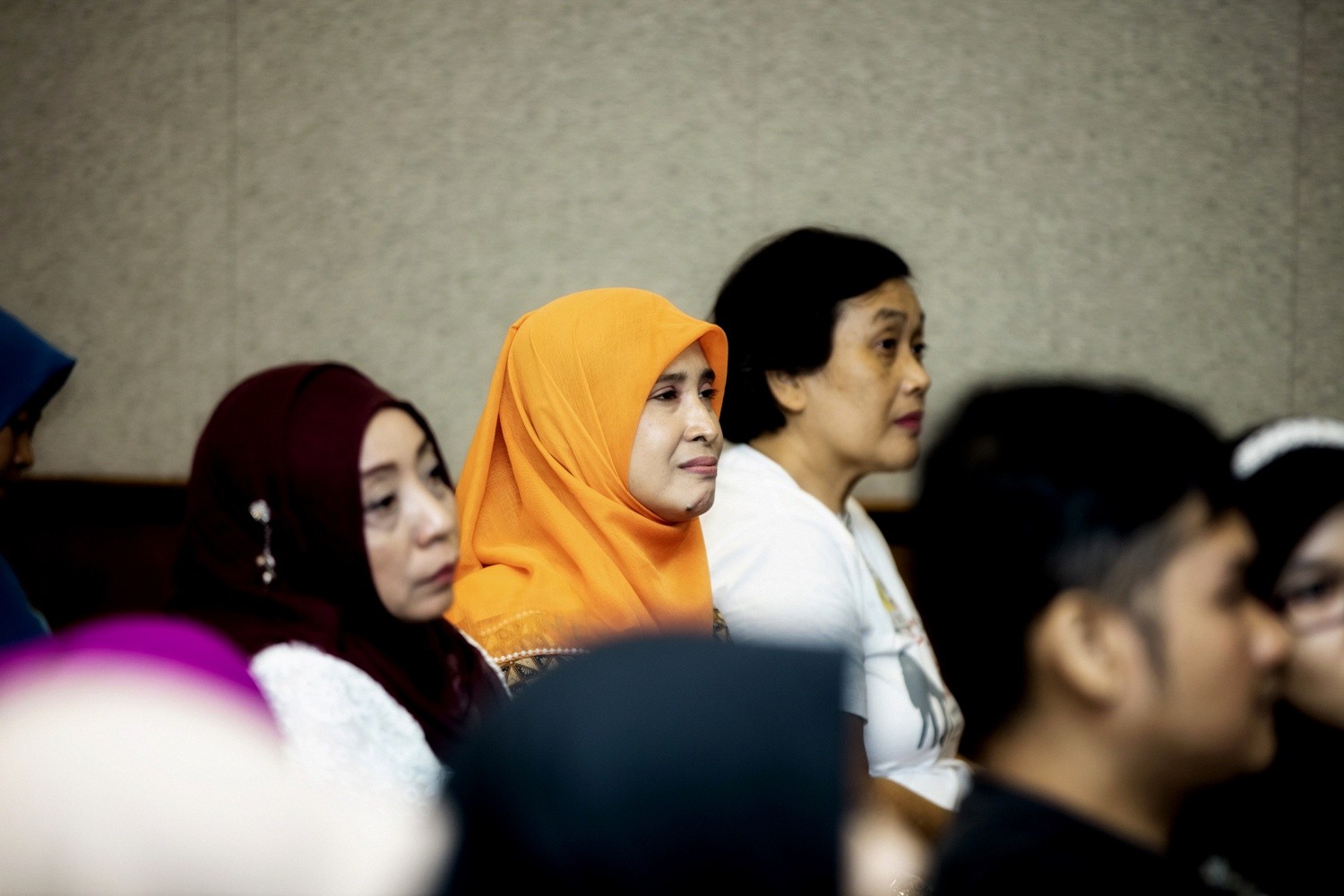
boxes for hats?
[1231,416,1344,606]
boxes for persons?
[155,364,520,782]
[1224,409,1344,896]
[451,287,958,849]
[0,310,61,654]
[910,363,1295,896]
[698,228,985,816]
[0,617,934,896]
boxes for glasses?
[1282,565,1344,625]
